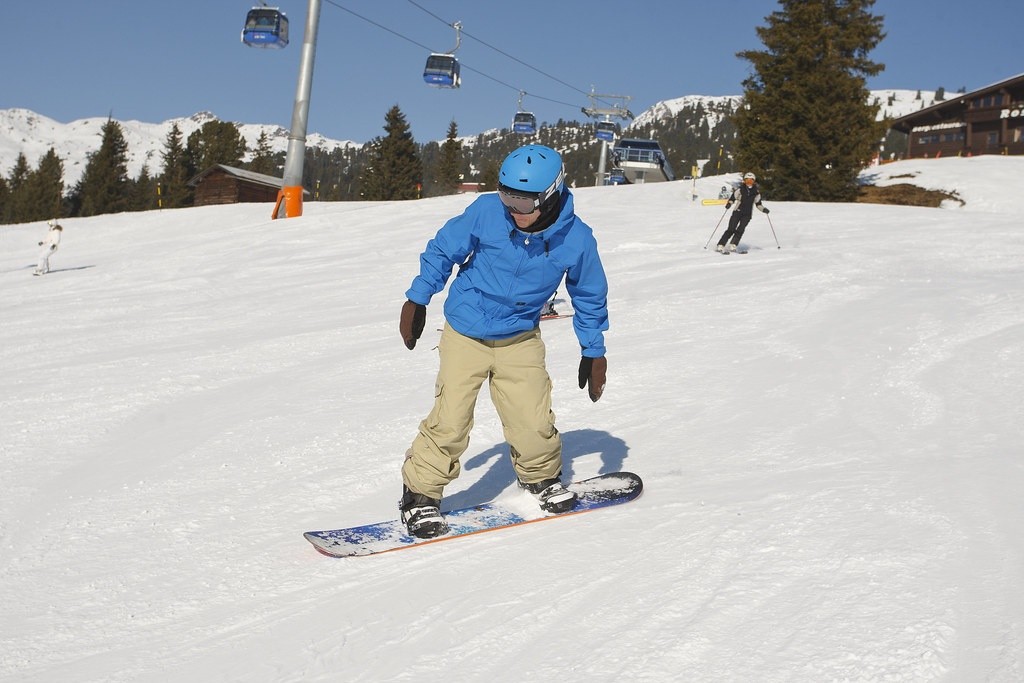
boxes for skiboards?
[716,246,749,254]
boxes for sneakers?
[517,471,578,513]
[398,482,449,539]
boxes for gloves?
[578,356,607,402]
[725,203,731,209]
[763,208,770,215]
[400,299,426,350]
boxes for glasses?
[496,183,535,214]
[745,179,754,184]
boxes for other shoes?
[717,244,725,252]
[728,243,737,251]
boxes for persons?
[398,145,608,539]
[715,172,770,252]
[34,218,62,275]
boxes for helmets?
[722,187,726,191]
[498,144,564,213]
[745,172,755,180]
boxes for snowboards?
[303,471,643,558]
